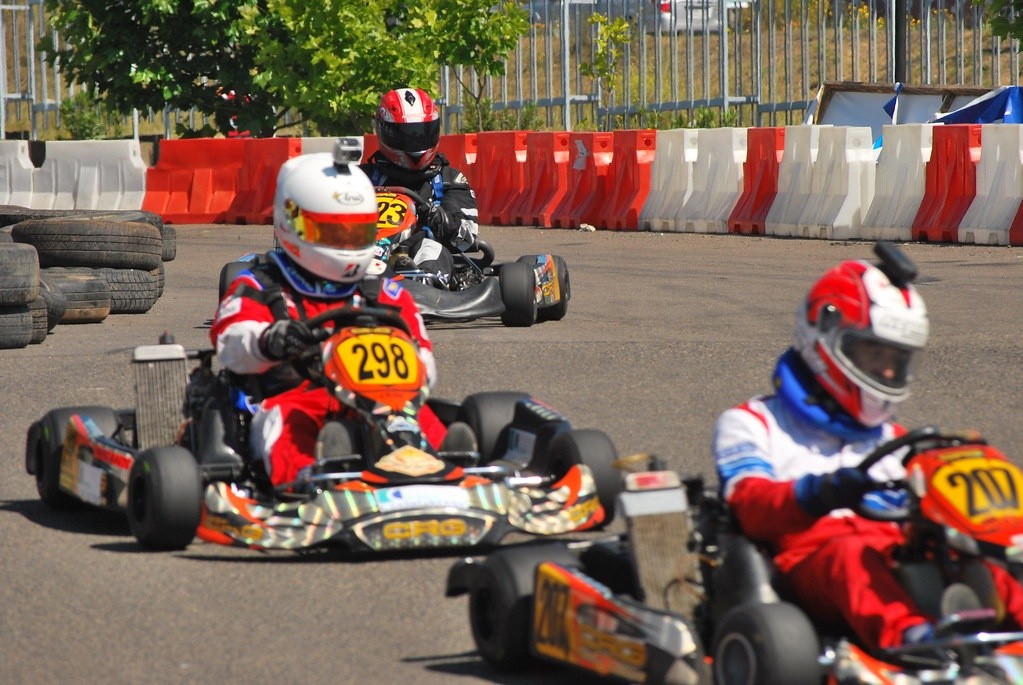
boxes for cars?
[549,1,749,36]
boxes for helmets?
[273,136,379,281]
[791,242,930,427]
[376,88,440,169]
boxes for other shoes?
[942,583,980,617]
[439,421,477,453]
[317,422,352,459]
[393,256,416,271]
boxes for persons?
[361,88,480,289]
[712,243,1023,684]
[209,151,477,478]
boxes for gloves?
[418,201,462,243]
[258,319,314,361]
[802,468,875,517]
